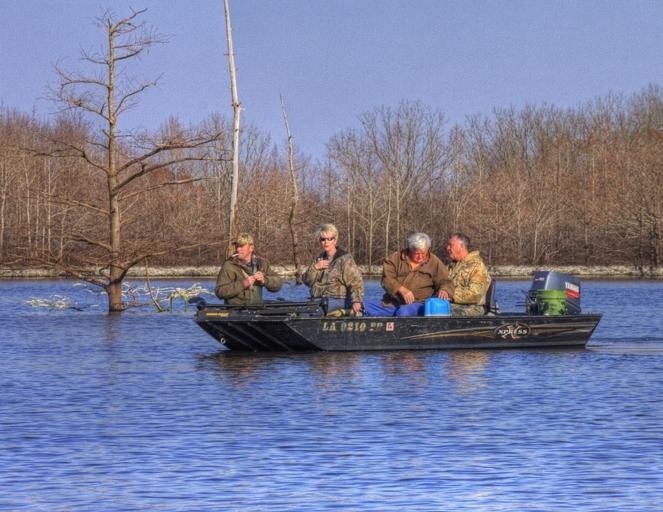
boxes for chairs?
[483,279,499,316]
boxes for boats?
[193,271,603,352]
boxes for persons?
[437,233,492,317]
[363,230,455,317]
[299,222,365,319]
[214,232,282,307]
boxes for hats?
[230,233,256,245]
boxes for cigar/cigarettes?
[231,254,238,258]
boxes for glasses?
[320,237,335,242]
